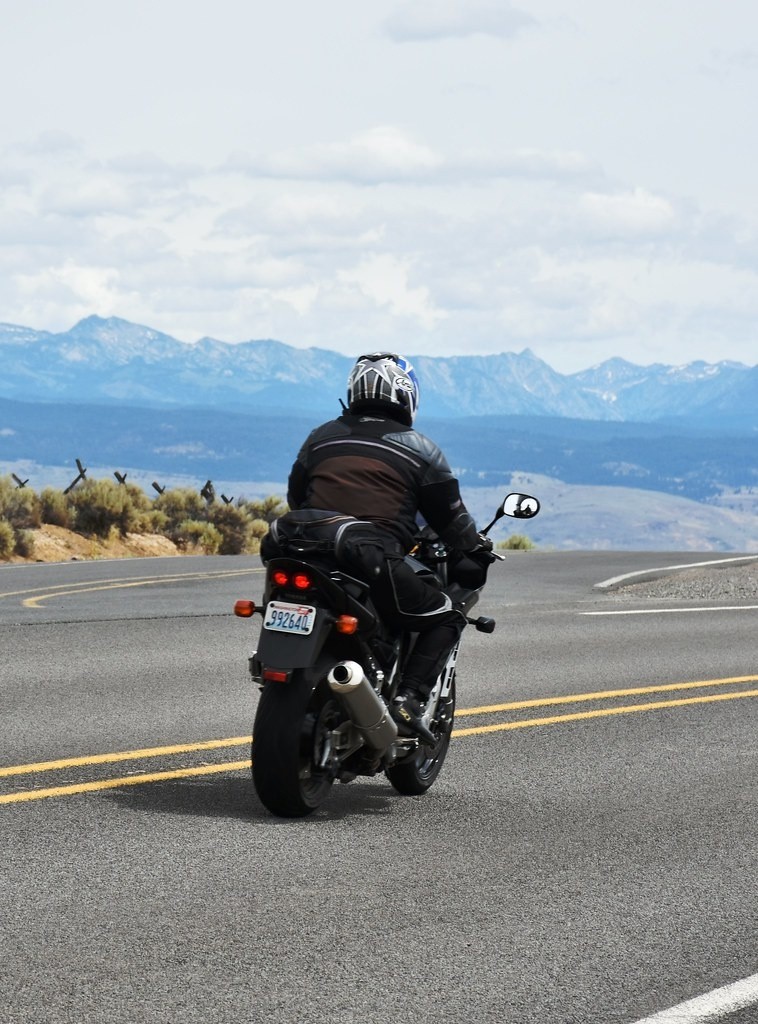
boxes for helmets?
[347,351,418,424]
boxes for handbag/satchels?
[259,509,387,583]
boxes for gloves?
[469,533,493,553]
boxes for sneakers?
[390,693,437,747]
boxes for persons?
[522,504,533,516]
[286,352,492,748]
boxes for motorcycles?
[235,493,542,820]
